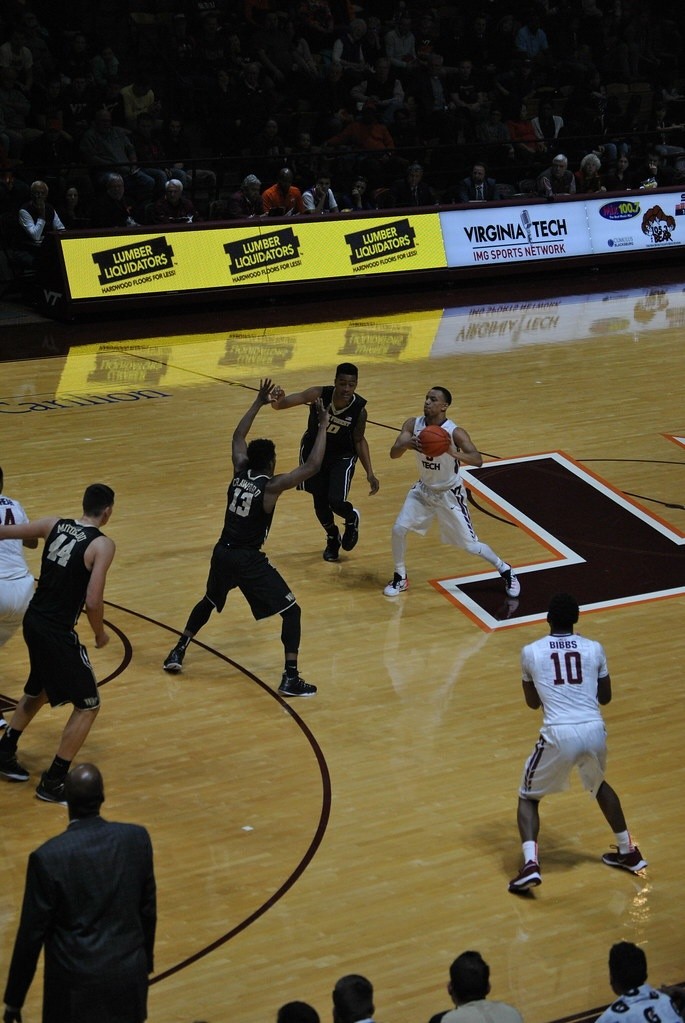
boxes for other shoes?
[0,715,6,728]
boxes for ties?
[477,186,483,201]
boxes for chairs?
[0,211,35,297]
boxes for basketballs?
[418,424,450,457]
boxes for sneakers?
[277,671,317,697]
[495,600,519,622]
[508,859,542,892]
[500,562,521,597]
[323,531,341,561]
[600,845,647,872]
[35,769,66,806]
[341,509,360,551]
[383,573,410,595]
[0,750,31,781]
[162,649,184,672]
[384,590,409,604]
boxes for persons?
[0,484,116,806]
[277,1001,321,1023]
[162,378,332,698]
[271,363,379,563]
[441,951,524,1023]
[0,467,39,651]
[0,0,685,286]
[510,593,648,890]
[0,758,158,1023]
[383,386,520,598]
[333,974,376,1023]
[594,941,683,1023]
